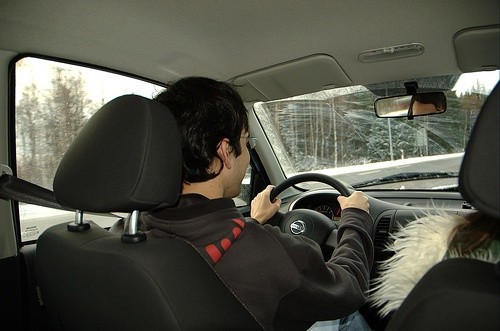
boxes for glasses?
[217,137,258,150]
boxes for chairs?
[376,79,500,331]
[30,94,264,331]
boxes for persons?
[409,92,446,115]
[363,197,500,318]
[108,75,375,331]
